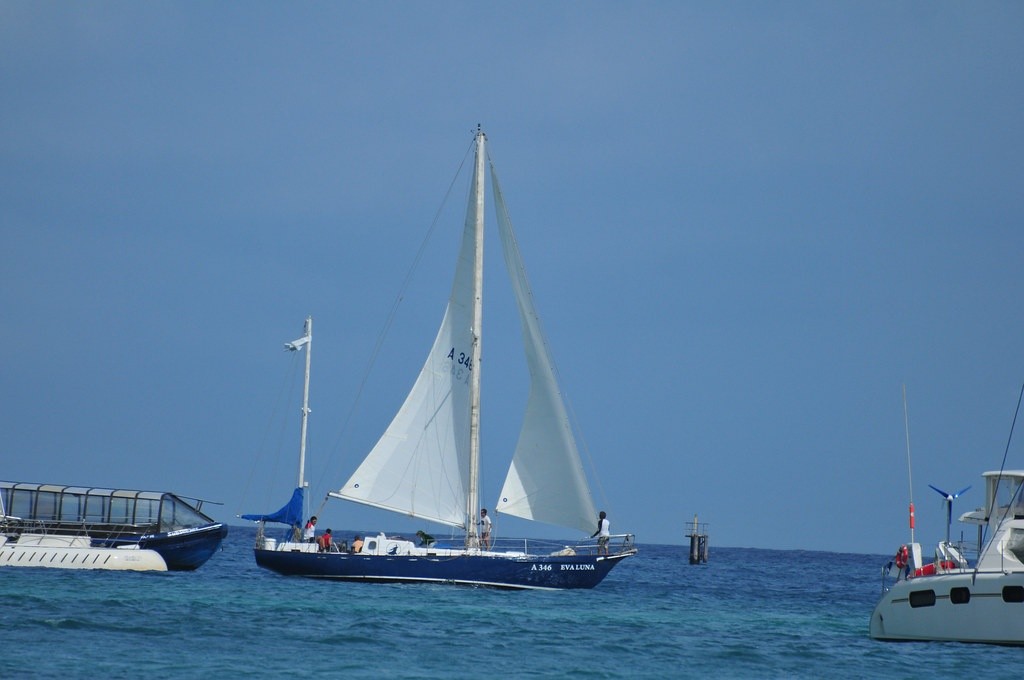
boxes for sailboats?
[235,122,643,591]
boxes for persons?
[303,516,317,543]
[477,508,492,550]
[416,530,438,548]
[588,510,610,555]
[323,528,332,552]
[351,535,363,552]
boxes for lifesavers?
[896,544,908,569]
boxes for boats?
[867,384,1023,648]
[0,479,231,572]
